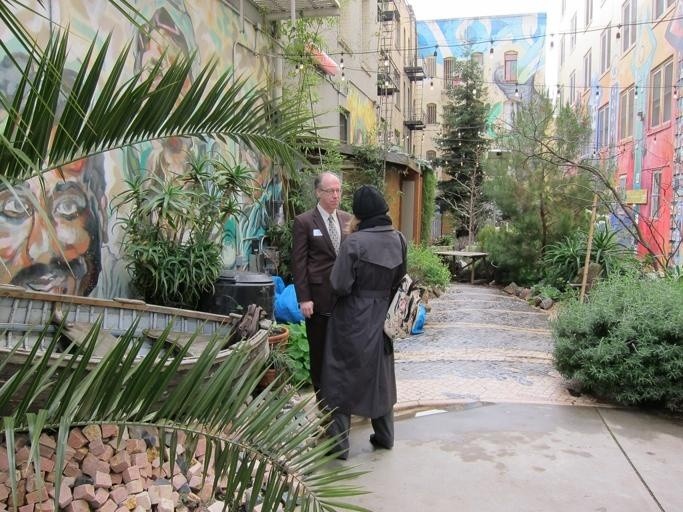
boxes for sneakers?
[367,432,392,449]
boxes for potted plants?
[259,341,297,388]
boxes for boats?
[0,284,272,414]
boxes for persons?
[131,6,194,134]
[289,170,353,439]
[0,51,107,299]
[316,185,409,462]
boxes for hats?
[350,183,391,220]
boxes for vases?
[268,327,289,353]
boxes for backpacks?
[382,274,428,341]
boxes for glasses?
[318,187,343,194]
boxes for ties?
[326,216,341,257]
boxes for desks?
[433,252,489,285]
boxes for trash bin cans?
[201,270,277,320]
